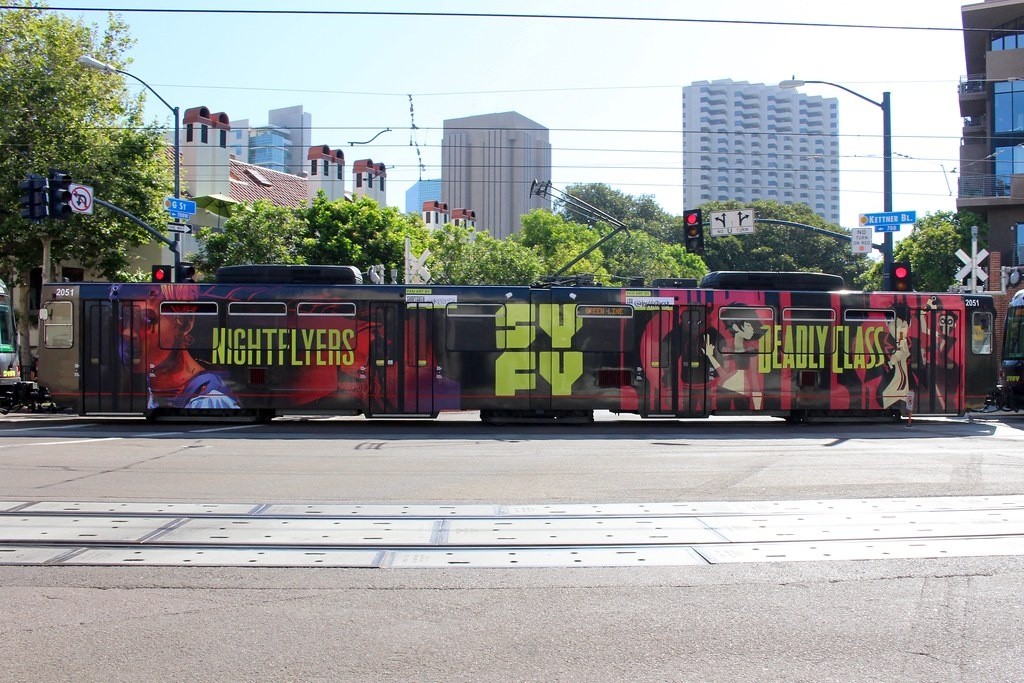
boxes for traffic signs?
[708,209,755,236]
[168,222,193,234]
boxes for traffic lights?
[683,208,704,253]
[177,261,194,283]
[19,178,47,221]
[891,262,913,291]
[48,168,71,220]
[152,265,171,283]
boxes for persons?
[30,358,38,381]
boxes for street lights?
[778,78,894,290]
[78,54,180,282]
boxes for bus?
[0,279,21,405]
[38,178,999,427]
[1002,289,1024,413]
